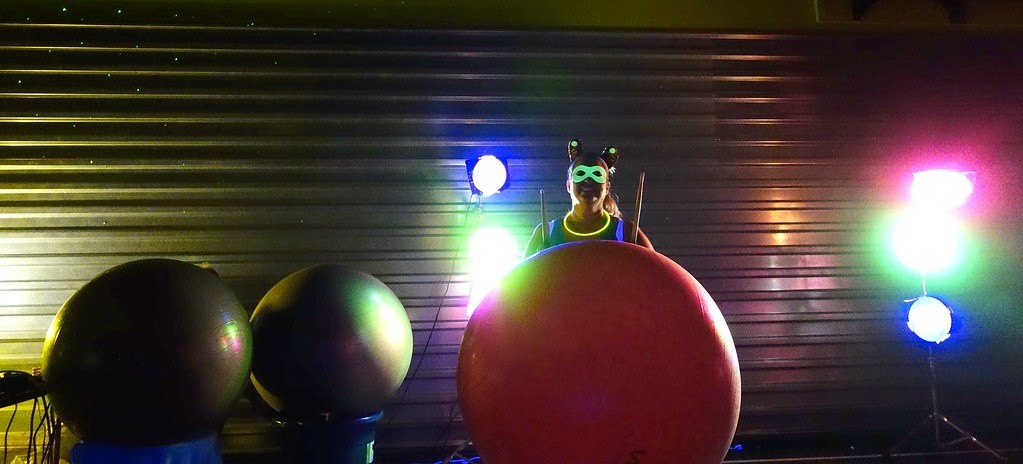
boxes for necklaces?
[563,210,610,236]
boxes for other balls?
[246,262,415,422]
[37,255,254,449]
[453,237,744,464]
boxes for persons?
[525,138,654,255]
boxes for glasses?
[568,165,610,184]
